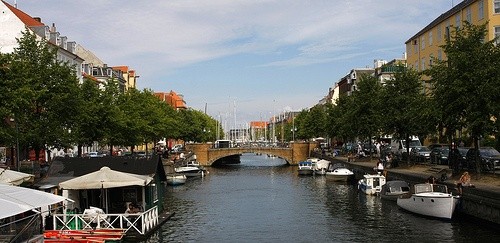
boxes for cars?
[447,147,472,169]
[409,146,431,163]
[55,143,184,158]
[429,146,450,165]
[465,146,500,174]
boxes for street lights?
[292,116,297,141]
[10,113,21,172]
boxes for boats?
[396,179,462,223]
[357,168,387,196]
[375,178,415,203]
[173,154,210,178]
[165,171,188,186]
[325,161,355,183]
[296,156,332,176]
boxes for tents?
[0,167,35,186]
[0,183,75,228]
[59,166,155,214]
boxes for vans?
[320,133,422,159]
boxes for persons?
[126,204,140,225]
